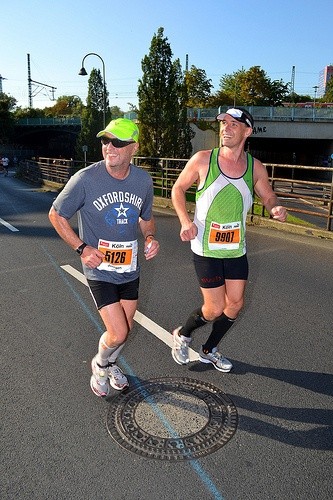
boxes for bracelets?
[146,235,155,240]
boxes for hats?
[215,107,252,127]
[96,118,139,142]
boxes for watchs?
[76,242,87,256]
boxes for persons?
[171,107,288,371]
[2,155,10,176]
[12,155,18,172]
[49,118,161,399]
[0,156,4,173]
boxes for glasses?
[100,136,136,148]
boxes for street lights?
[233,76,257,108]
[312,85,319,121]
[78,52,106,131]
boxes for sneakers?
[107,365,130,391]
[90,357,110,397]
[198,345,232,372]
[172,326,193,365]
